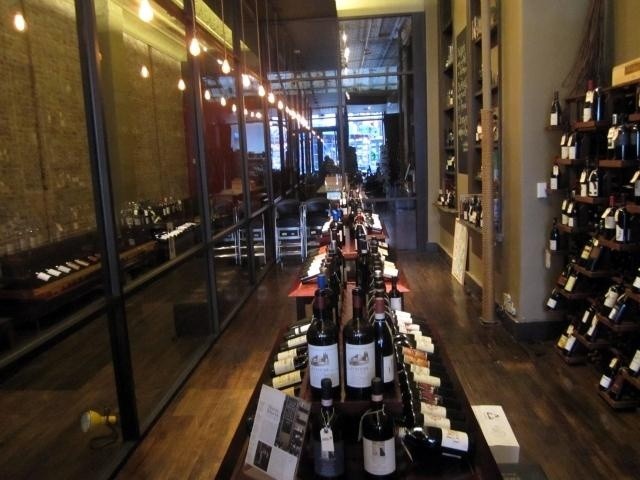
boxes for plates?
[542,78,640,410]
[433,1,505,245]
[121,215,200,259]
[1,237,160,333]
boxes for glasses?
[80,405,120,451]
[13,0,27,32]
[138,0,325,145]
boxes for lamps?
[138,0,325,145]
[210,198,267,264]
[13,0,27,32]
[275,197,334,263]
[80,405,120,451]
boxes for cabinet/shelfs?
[367,169,371,177]
[438,87,483,228]
[1,237,160,333]
[215,211,503,480]
[121,215,200,259]
[542,78,640,410]
[433,1,505,245]
[27,197,198,283]
[546,80,640,392]
[0,205,96,257]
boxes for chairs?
[275,197,334,263]
[210,198,267,264]
